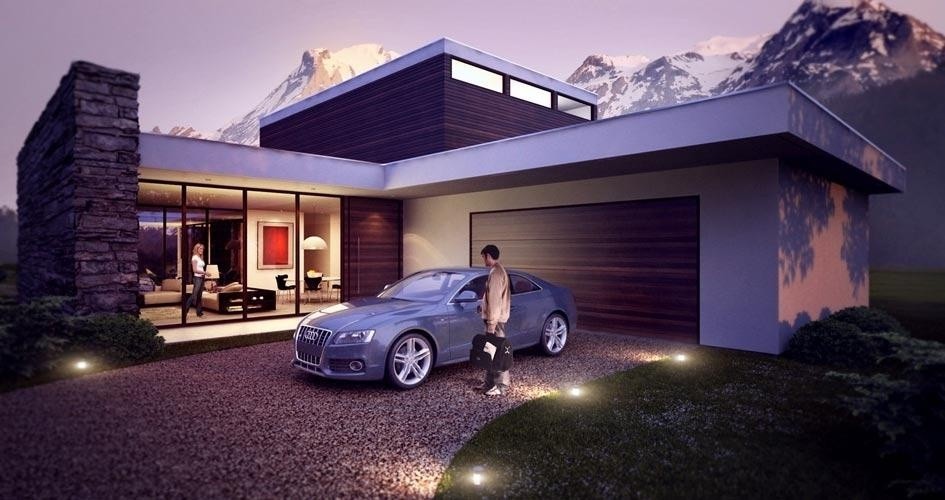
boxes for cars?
[289,266,580,392]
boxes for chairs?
[275,270,341,305]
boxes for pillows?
[162,279,182,291]
[223,282,239,290]
[224,285,243,291]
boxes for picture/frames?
[256,221,294,270]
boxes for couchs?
[140,285,276,314]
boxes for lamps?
[205,265,220,293]
[304,205,328,250]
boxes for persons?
[478,244,511,397]
[186,243,212,317]
